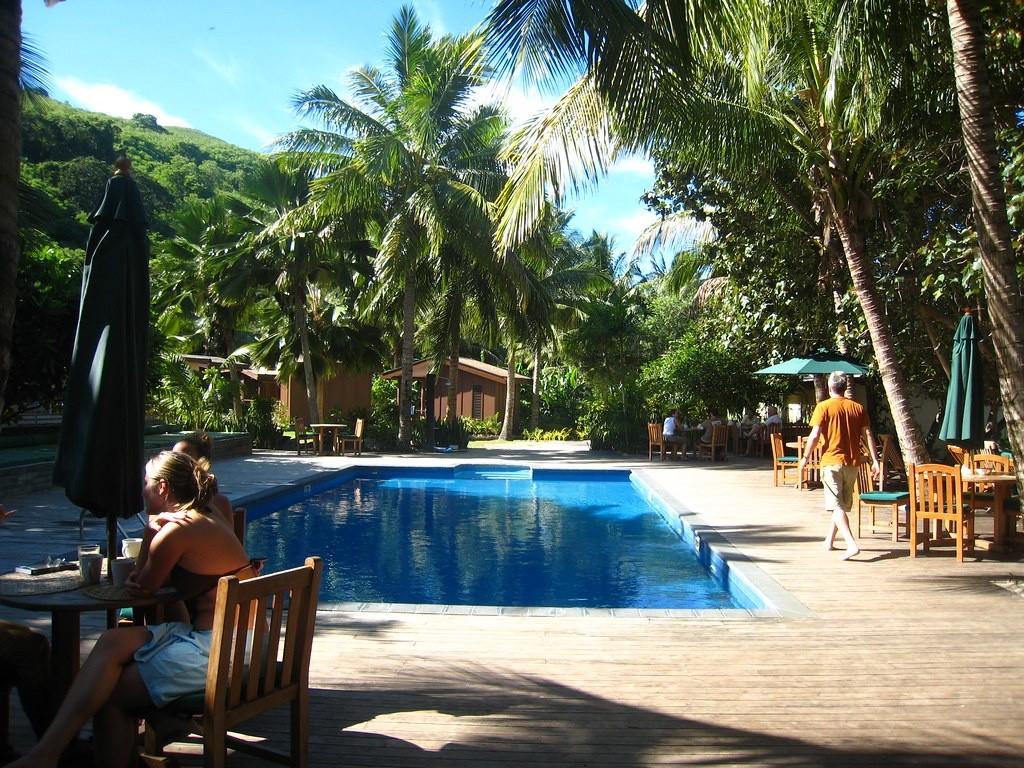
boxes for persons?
[740,406,782,457]
[696,407,728,462]
[0,435,274,768]
[662,409,691,461]
[798,371,880,562]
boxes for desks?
[678,428,703,459]
[925,473,1017,553]
[787,442,824,488]
[0,557,182,768]
[310,424,347,456]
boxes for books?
[15,561,77,575]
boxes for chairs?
[338,418,364,456]
[294,418,319,455]
[118,508,323,768]
[647,422,1024,563]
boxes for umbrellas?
[752,347,877,375]
[51,155,152,629]
[937,307,984,556]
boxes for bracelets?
[802,454,809,460]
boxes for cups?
[111,559,135,587]
[79,554,103,585]
[78,544,100,558]
[121,538,142,558]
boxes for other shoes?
[739,452,752,456]
[672,452,681,458]
[681,455,691,461]
